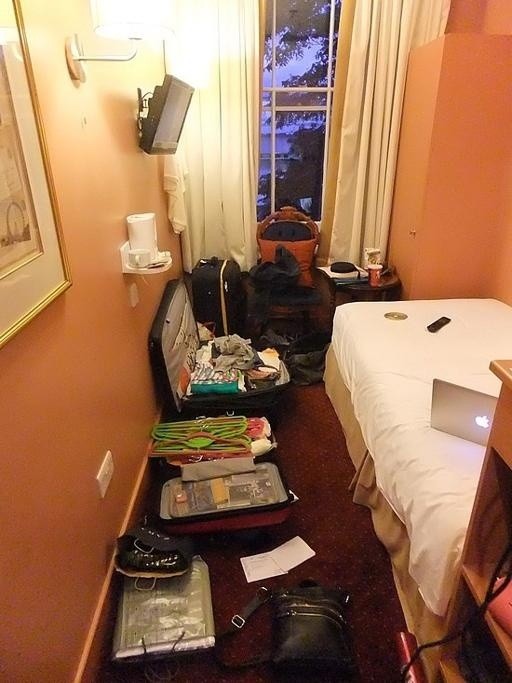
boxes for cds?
[384,312,408,320]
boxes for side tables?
[326,273,401,342]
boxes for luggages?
[190,254,246,342]
[151,411,299,537]
[147,276,294,429]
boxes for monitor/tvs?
[138,73,196,155]
[430,376,499,447]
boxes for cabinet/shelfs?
[431,359,512,682]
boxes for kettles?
[126,212,158,265]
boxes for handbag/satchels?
[116,521,195,593]
[110,552,218,682]
[195,319,218,341]
[269,577,358,679]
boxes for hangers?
[146,413,255,461]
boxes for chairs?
[254,205,321,340]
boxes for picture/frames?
[0,0,72,346]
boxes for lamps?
[66,0,177,81]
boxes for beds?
[324,296,512,681]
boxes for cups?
[128,248,151,268]
[368,264,383,287]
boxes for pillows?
[257,237,318,290]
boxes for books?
[168,476,229,516]
[223,477,280,506]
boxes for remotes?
[427,316,451,333]
